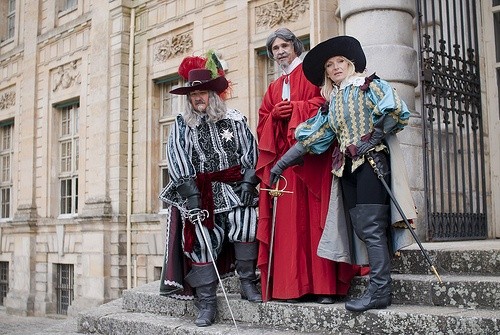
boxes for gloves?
[178,178,202,223]
[269,144,305,186]
[271,99,292,121]
[357,115,405,155]
[234,169,261,205]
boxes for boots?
[234,242,264,303]
[184,263,218,327]
[345,204,391,312]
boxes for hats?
[169,48,228,95]
[302,36,366,87]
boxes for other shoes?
[318,294,336,304]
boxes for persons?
[158,69,262,327]
[254,29,351,304]
[269,36,411,312]
